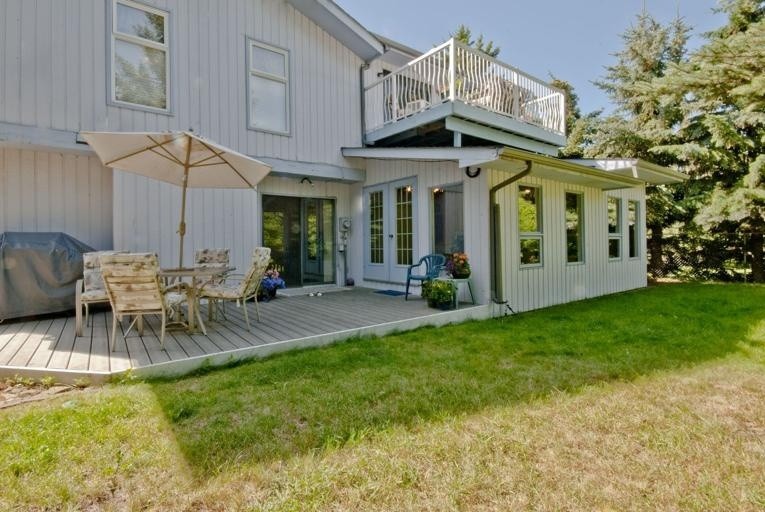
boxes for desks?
[154,268,236,334]
[438,278,475,309]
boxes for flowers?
[448,253,471,269]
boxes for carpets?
[371,289,413,297]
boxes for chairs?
[189,249,230,321]
[405,253,444,302]
[73,249,131,338]
[200,245,272,331]
[98,251,195,352]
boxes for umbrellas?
[79,125,275,324]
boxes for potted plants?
[437,281,458,309]
[423,280,439,308]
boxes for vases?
[450,269,470,278]
